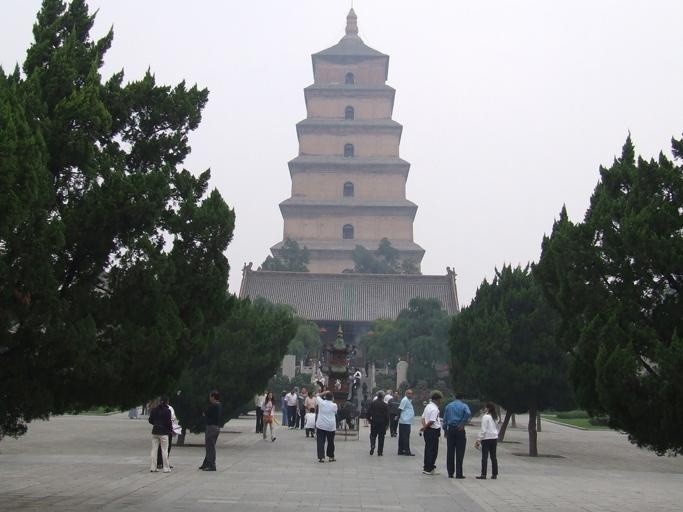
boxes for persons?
[281,379,344,463]
[442,393,471,478]
[475,403,499,479]
[418,390,441,474]
[199,390,222,471]
[156,402,182,469]
[255,388,277,441]
[149,395,171,473]
[128,400,155,420]
[352,361,415,456]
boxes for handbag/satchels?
[263,416,273,423]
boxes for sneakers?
[449,474,497,479]
[319,459,324,462]
[378,452,383,456]
[151,464,174,473]
[422,468,440,475]
[329,458,336,462]
[370,445,375,455]
[398,450,415,456]
[199,466,216,471]
[272,437,276,442]
